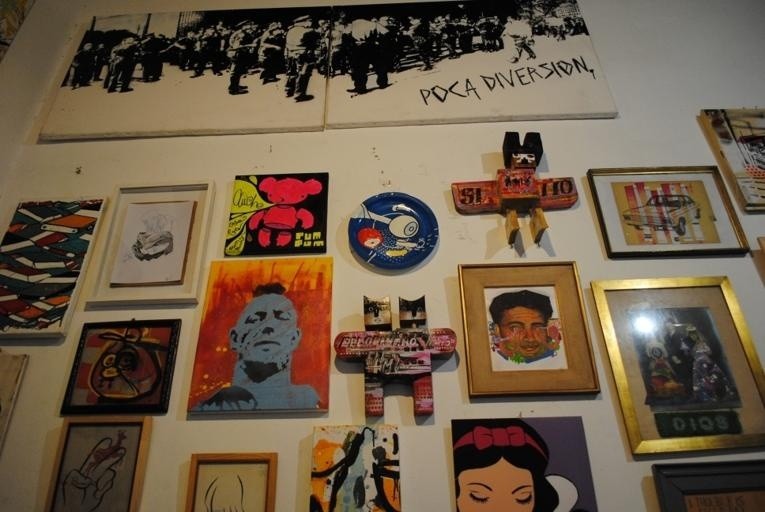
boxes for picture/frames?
[84,180,216,304]
[2,196,108,338]
[650,459,765,512]
[699,107,765,212]
[42,416,154,512]
[586,164,751,261]
[184,452,278,512]
[590,274,765,460]
[456,260,601,397]
[58,318,184,415]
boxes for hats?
[236,19,249,29]
[293,14,312,23]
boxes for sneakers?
[227,85,248,95]
[285,91,313,102]
[346,83,387,94]
[509,55,537,64]
[61,75,163,93]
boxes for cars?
[620,189,705,236]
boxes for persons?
[485,283,563,368]
[190,282,322,410]
[648,309,699,400]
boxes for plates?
[349,193,438,269]
